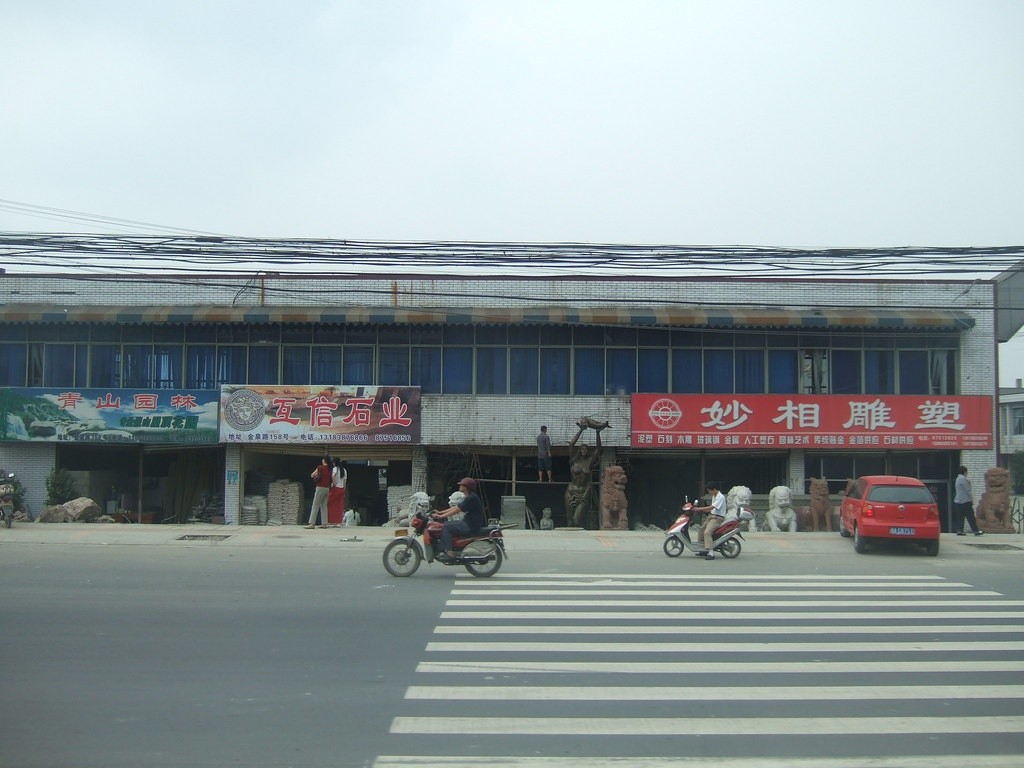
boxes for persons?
[540,508,553,530]
[537,426,552,482]
[693,480,726,560]
[431,478,484,557]
[304,457,360,529]
[954,466,983,535]
[564,421,602,527]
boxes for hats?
[458,478,477,491]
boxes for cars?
[839,475,941,555]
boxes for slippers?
[437,553,453,560]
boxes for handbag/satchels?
[314,466,322,481]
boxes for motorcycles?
[383,495,518,578]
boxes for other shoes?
[304,525,315,529]
[957,532,966,535]
[695,552,708,556]
[975,531,985,535]
[705,555,714,560]
[318,526,328,529]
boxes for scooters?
[0,473,15,528]
[663,495,745,558]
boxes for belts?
[713,514,723,518]
